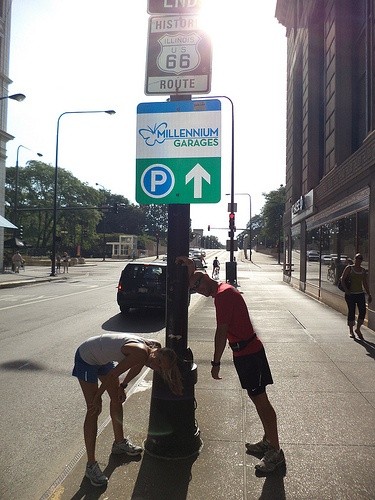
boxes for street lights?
[51,109,115,276]
[14,144,44,237]
[226,193,251,261]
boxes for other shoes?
[356,329,363,339]
[349,330,354,338]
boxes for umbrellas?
[4,236,33,251]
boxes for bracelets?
[211,360,220,367]
[120,383,126,389]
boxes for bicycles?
[327,267,335,283]
[215,266,219,279]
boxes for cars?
[192,250,202,262]
[306,249,352,265]
[194,258,208,274]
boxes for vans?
[115,259,168,314]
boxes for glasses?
[354,256,363,260]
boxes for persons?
[211,256,220,278]
[12,250,21,274]
[62,252,69,273]
[56,255,61,274]
[340,253,373,339]
[70,333,182,483]
[174,255,286,473]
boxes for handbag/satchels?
[229,333,257,351]
[338,276,351,292]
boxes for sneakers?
[85,460,109,486]
[245,434,269,453]
[112,437,143,456]
[255,448,285,473]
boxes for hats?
[187,270,207,294]
